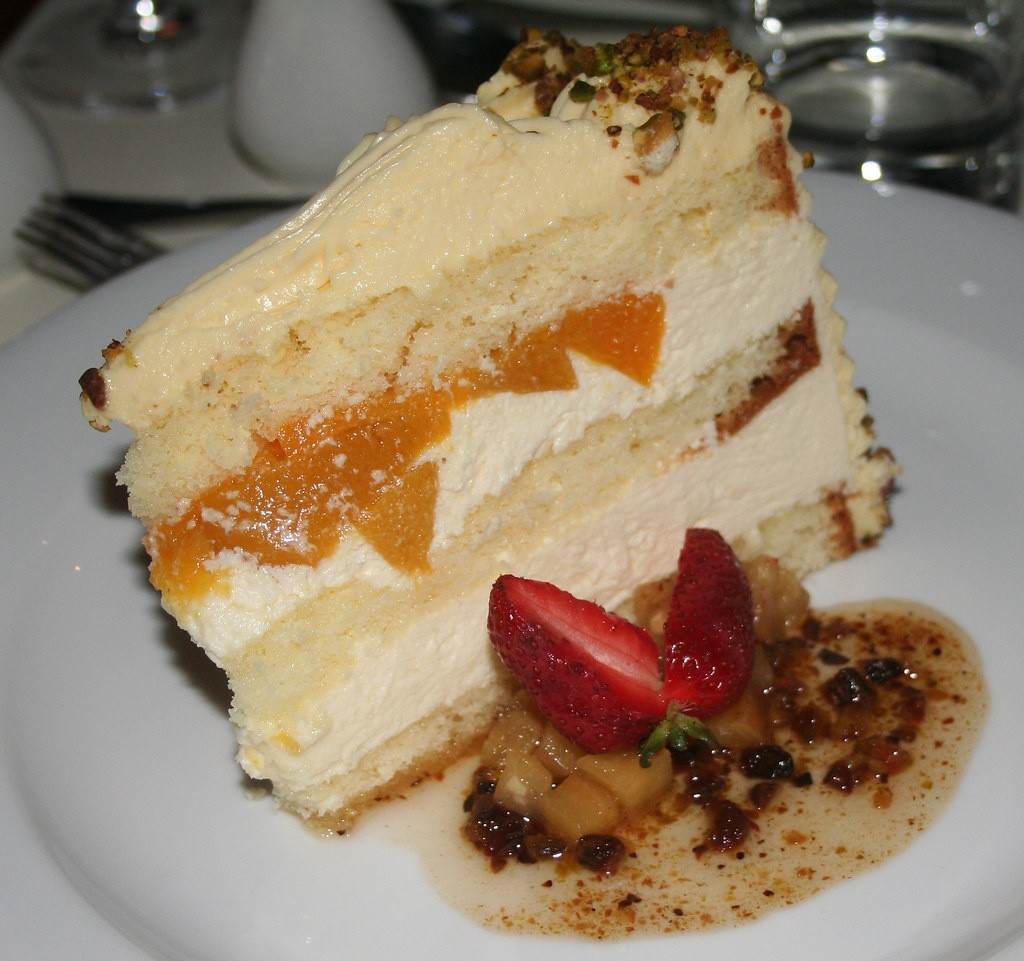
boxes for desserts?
[76,25,896,837]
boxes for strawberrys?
[484,526,755,764]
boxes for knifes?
[38,190,314,227]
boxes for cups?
[716,0,1024,204]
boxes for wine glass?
[11,0,250,107]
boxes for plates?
[1,168,1024,961]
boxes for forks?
[15,189,167,286]
